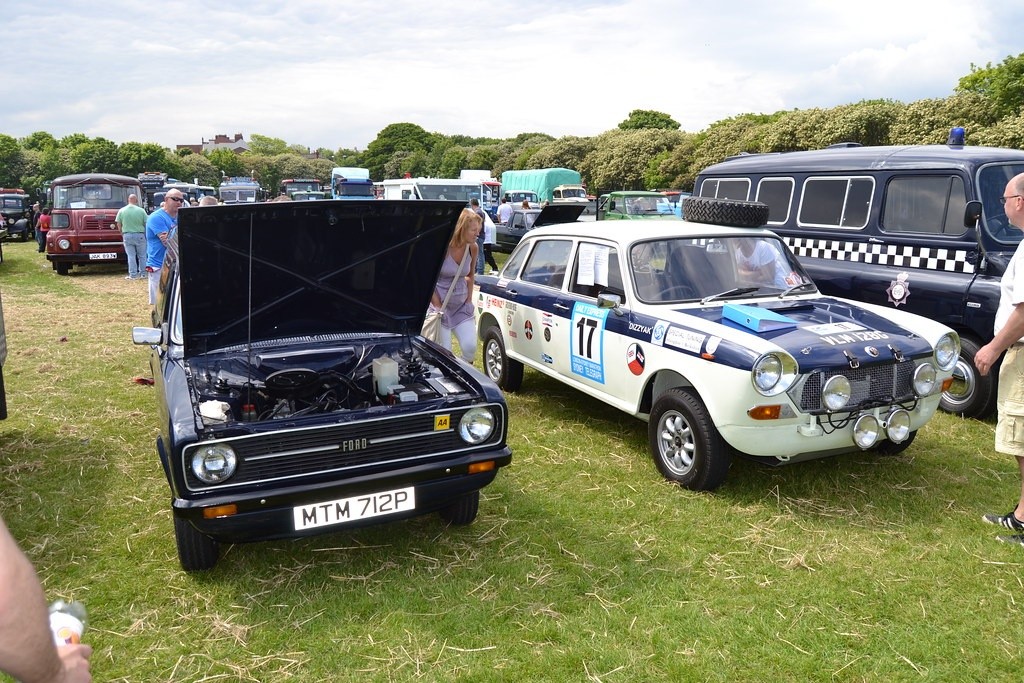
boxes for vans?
[690,127,1024,421]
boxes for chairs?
[105,201,125,208]
[612,271,660,302]
[666,246,725,302]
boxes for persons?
[33,204,51,253]
[521,200,530,210]
[115,194,148,280]
[497,199,513,223]
[974,173,1024,547]
[146,188,226,305]
[0,512,91,683]
[546,202,549,206]
[470,199,500,275]
[734,238,793,288]
[430,208,482,364]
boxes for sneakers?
[982,503,1024,532]
[996,534,1024,549]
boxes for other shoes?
[125,276,138,281]
[140,274,146,279]
[489,270,500,276]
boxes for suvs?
[0,188,35,242]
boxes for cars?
[471,217,962,495]
[133,198,513,577]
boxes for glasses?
[166,196,184,203]
[998,194,1024,204]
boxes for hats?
[219,198,224,202]
[470,198,480,206]
[42,208,49,214]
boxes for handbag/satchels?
[420,311,444,346]
[42,223,49,228]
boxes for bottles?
[47,599,89,651]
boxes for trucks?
[45,167,686,275]
